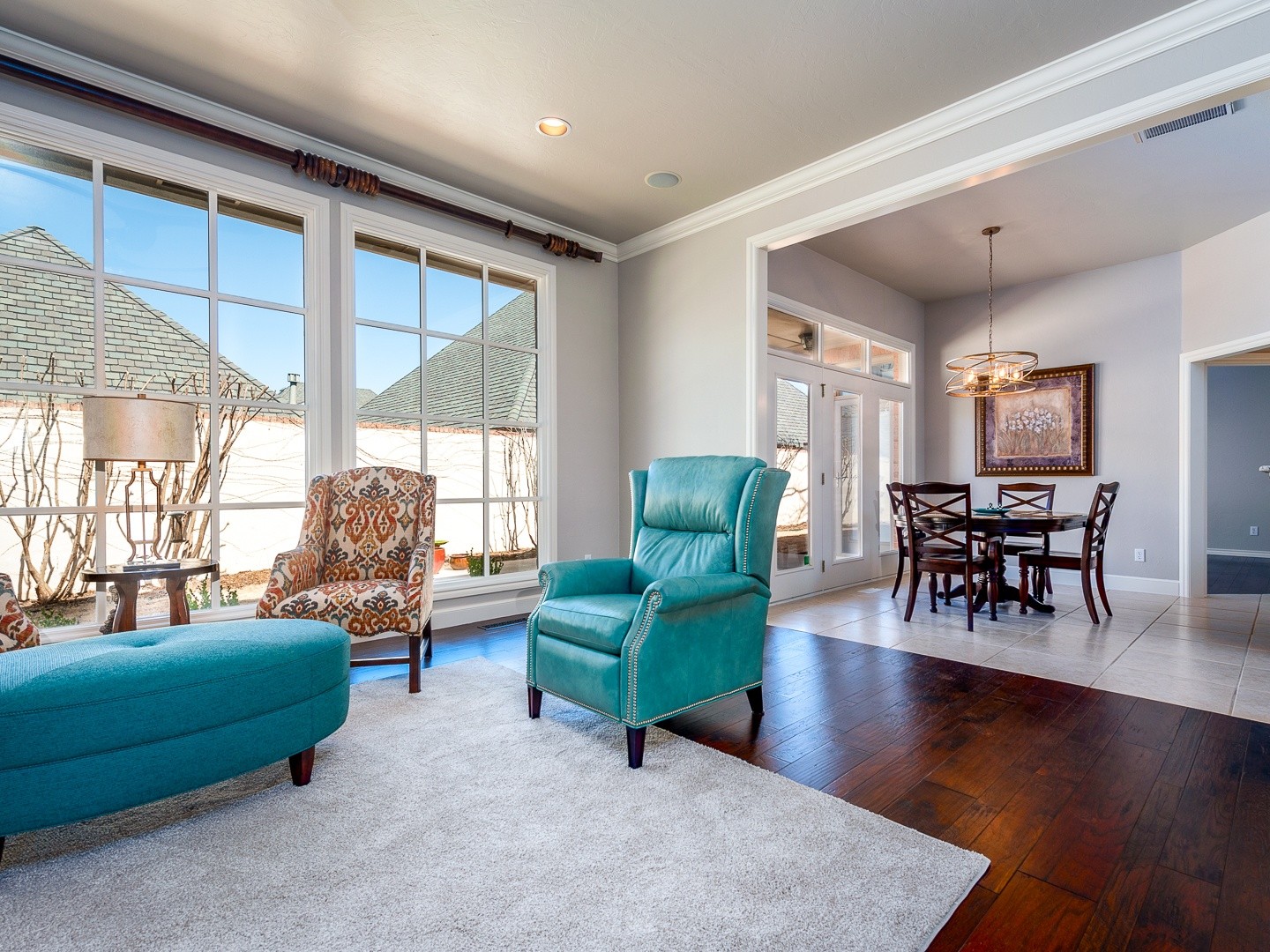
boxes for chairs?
[886,482,969,599]
[255,467,437,693]
[900,482,990,632]
[977,483,1056,599]
[525,455,791,770]
[1018,482,1121,625]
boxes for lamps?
[946,226,1038,397]
[798,333,812,352]
[81,394,196,571]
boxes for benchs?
[0,619,352,864]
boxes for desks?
[892,511,1090,614]
[81,559,218,633]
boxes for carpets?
[0,656,991,952]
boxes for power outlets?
[1250,526,1258,535]
[1135,549,1145,561]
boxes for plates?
[972,508,1012,515]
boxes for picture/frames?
[974,363,1095,476]
